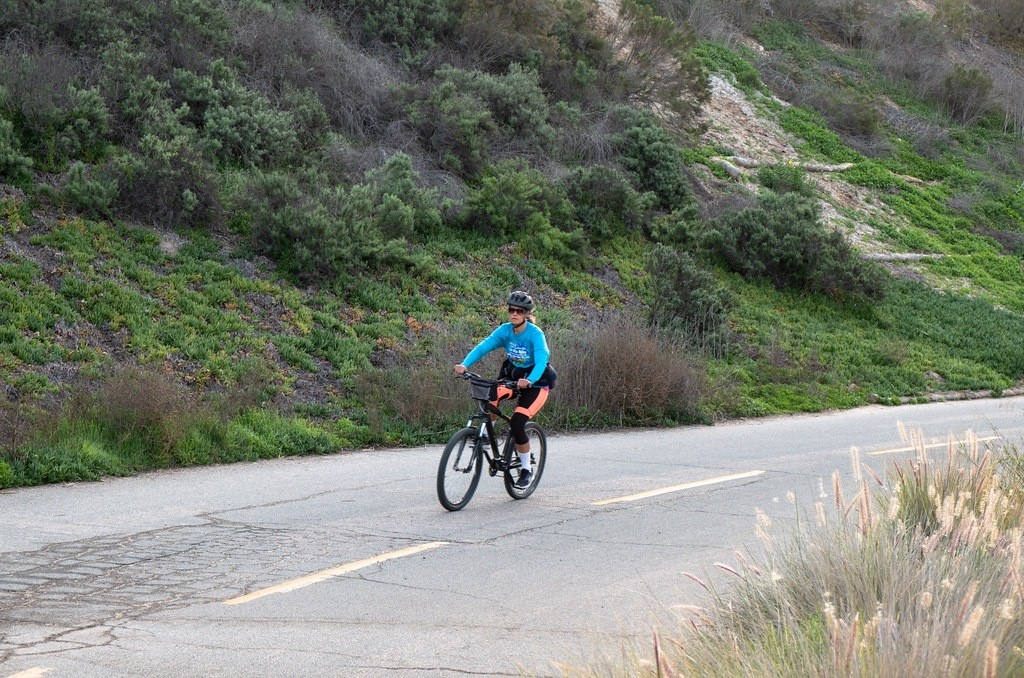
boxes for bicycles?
[437,366,547,512]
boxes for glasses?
[509,308,527,314]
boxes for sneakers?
[469,437,491,448]
[514,467,535,489]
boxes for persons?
[454,291,557,489]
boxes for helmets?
[507,291,533,311]
